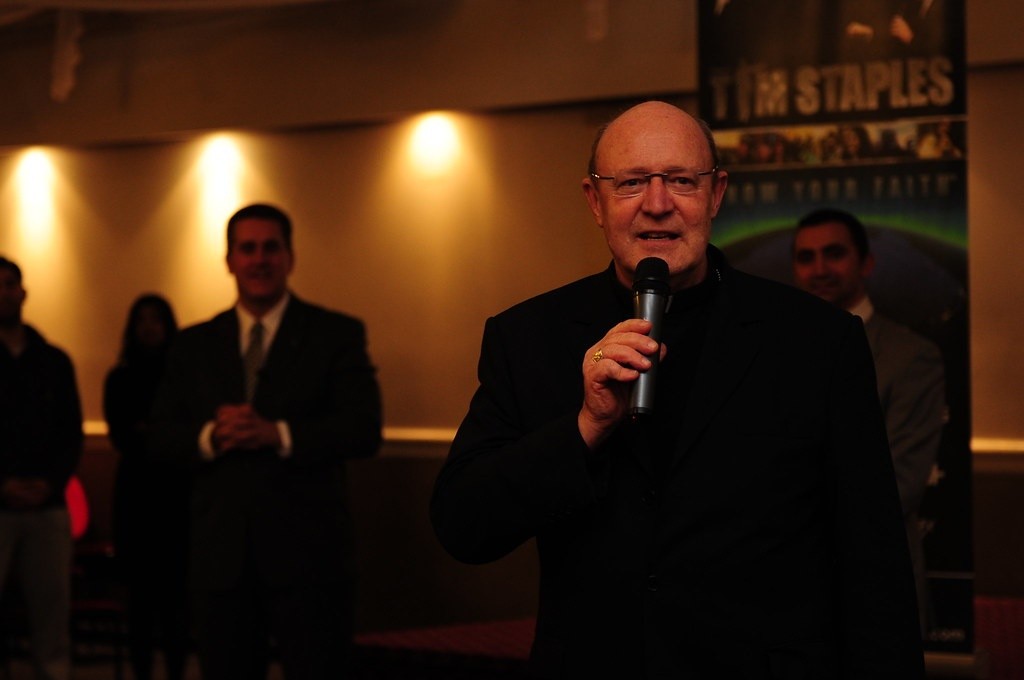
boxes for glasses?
[587,167,717,197]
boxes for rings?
[594,349,602,361]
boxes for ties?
[242,320,266,408]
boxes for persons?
[0,256,85,680]
[149,204,384,680]
[431,102,925,680]
[792,209,945,641]
[100,293,193,680]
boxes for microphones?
[628,257,670,417]
